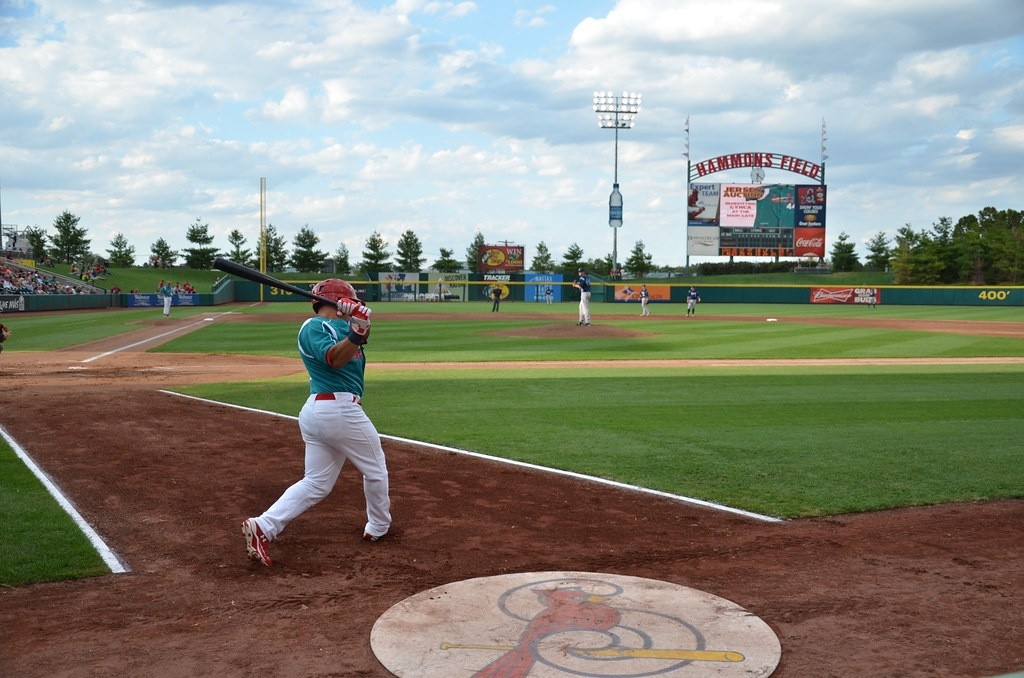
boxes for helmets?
[311,278,366,314]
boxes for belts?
[315,393,361,405]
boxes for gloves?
[336,298,356,316]
[348,302,372,345]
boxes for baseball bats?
[212,257,338,308]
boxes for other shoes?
[576,322,585,326]
[692,314,694,317]
[640,314,645,316]
[584,322,591,326]
[686,314,688,317]
[646,313,650,316]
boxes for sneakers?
[363,532,378,542]
[241,517,272,566]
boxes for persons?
[546,287,552,304]
[868,290,875,308]
[0,252,96,294]
[72,265,112,282]
[0,323,9,354]
[241,278,394,567]
[573,268,592,326]
[610,268,622,280]
[492,285,502,312]
[686,286,700,317]
[111,284,121,293]
[158,280,172,316]
[130,288,141,293]
[173,281,196,293]
[638,285,650,316]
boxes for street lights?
[593,89,642,282]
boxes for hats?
[577,268,584,272]
